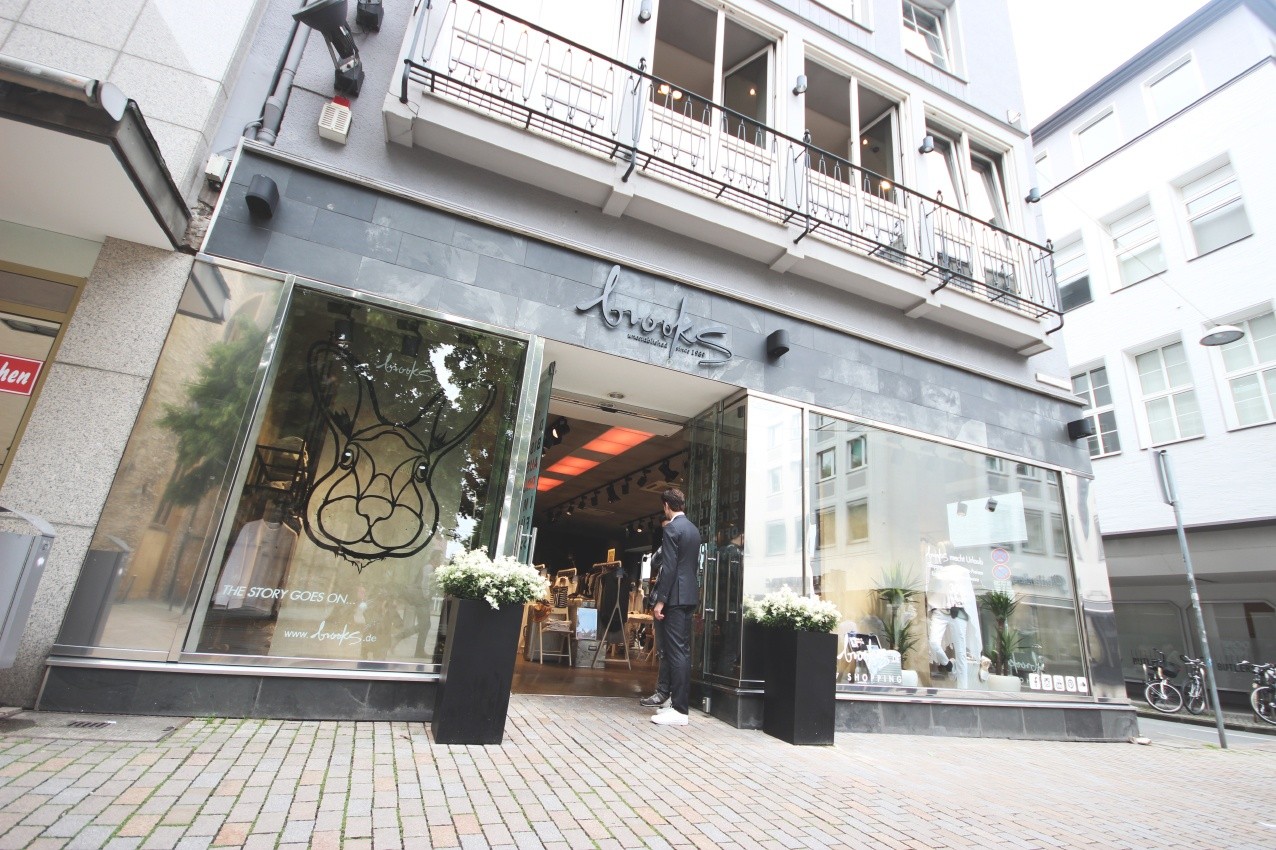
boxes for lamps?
[327,301,353,343]
[1068,418,1092,441]
[1024,188,1041,204]
[456,332,485,371]
[767,329,790,359]
[543,447,691,524]
[791,74,808,96]
[1198,323,1245,347]
[396,318,423,359]
[290,0,367,98]
[637,0,652,23]
[243,172,280,218]
[539,415,569,458]
[918,135,935,154]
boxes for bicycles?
[1179,654,1208,715]
[1143,648,1184,712]
[1236,660,1276,725]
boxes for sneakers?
[657,707,674,715]
[650,708,689,725]
[641,692,674,708]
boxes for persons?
[926,553,972,688]
[714,523,743,689]
[639,490,702,724]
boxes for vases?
[431,595,525,744]
[764,629,842,746]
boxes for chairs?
[531,605,573,667]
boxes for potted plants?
[867,556,921,687]
[976,580,1027,692]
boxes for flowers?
[744,582,842,634]
[431,548,551,610]
